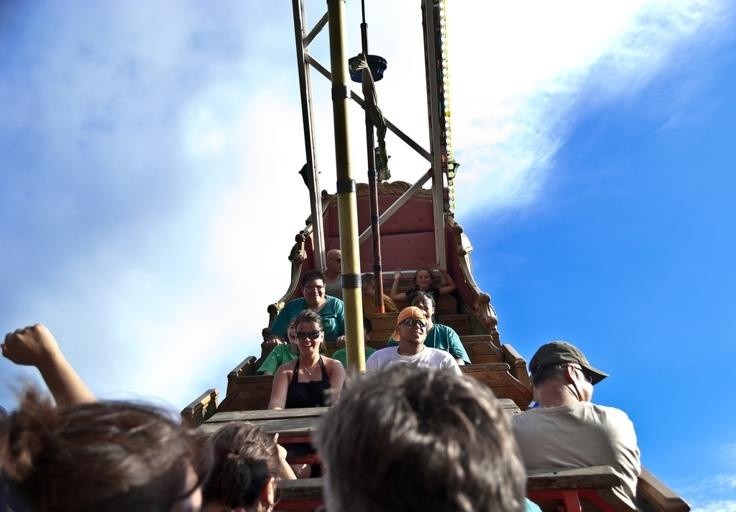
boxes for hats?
[392,306,427,341]
[529,341,609,385]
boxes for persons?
[255,316,324,376]
[320,248,342,301]
[331,316,377,369]
[0,323,283,511]
[308,364,542,511]
[361,273,400,314]
[384,291,473,366]
[512,338,644,499]
[266,309,346,478]
[265,269,345,346]
[391,265,457,315]
[366,305,463,374]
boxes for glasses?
[304,285,324,289]
[561,365,592,384]
[402,319,427,327]
[296,329,324,340]
